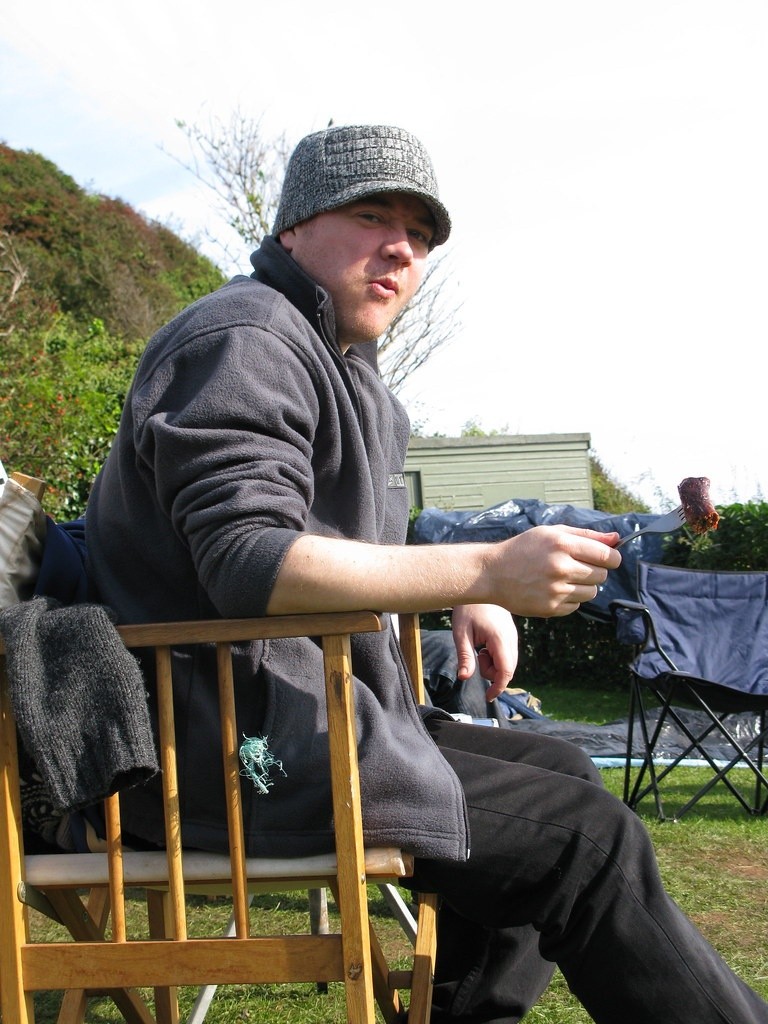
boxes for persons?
[81,125,768,1024]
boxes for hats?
[271,125,452,255]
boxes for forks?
[610,503,687,550]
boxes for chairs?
[609,559,767,823]
[1,472,440,1023]
